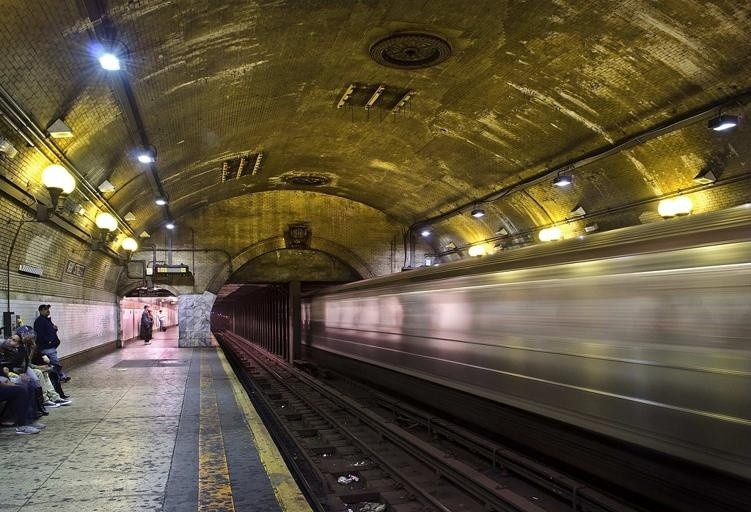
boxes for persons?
[0,304,72,435]
[158,310,166,332]
[142,305,154,345]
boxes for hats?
[38,305,50,311]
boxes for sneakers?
[15,419,47,434]
[44,398,71,408]
[33,407,49,418]
